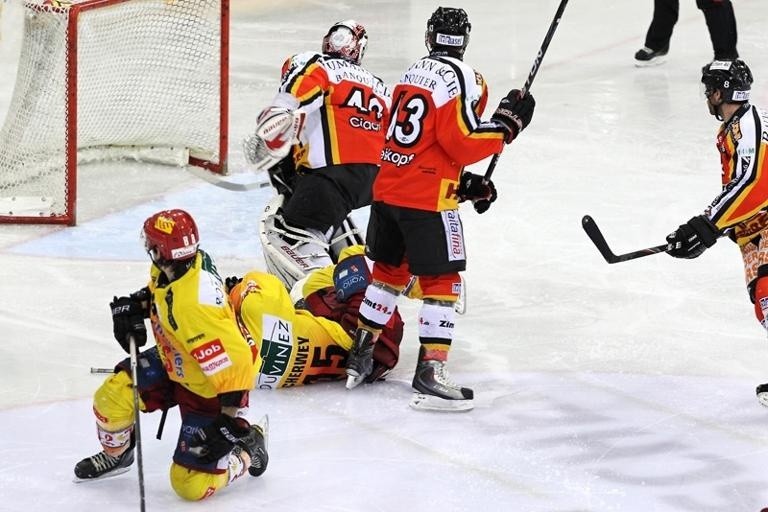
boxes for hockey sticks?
[190,164,271,191]
[583,216,681,262]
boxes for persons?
[224,244,404,388]
[668,60,767,405]
[634,0,738,68]
[74,210,269,502]
[344,6,535,411]
[267,51,333,200]
[267,16,393,281]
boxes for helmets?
[321,20,368,65]
[140,209,199,263]
[278,52,316,88]
[425,6,470,60]
[700,59,753,104]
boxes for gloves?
[109,296,147,357]
[457,172,496,213]
[490,89,535,144]
[665,215,723,260]
[187,413,250,463]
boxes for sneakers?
[756,382,768,403]
[634,45,669,61]
[245,425,268,477]
[74,451,134,479]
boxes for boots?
[411,360,472,402]
[346,328,373,376]
